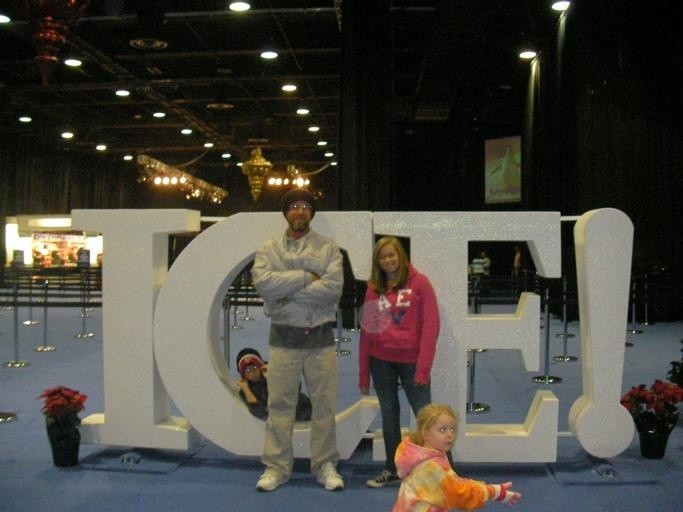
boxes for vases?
[31,385,87,433]
[620,379,682,432]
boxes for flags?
[638,427,668,459]
[47,431,80,466]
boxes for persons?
[234,347,312,419]
[251,186,346,491]
[389,401,521,510]
[356,233,442,489]
[469,245,528,295]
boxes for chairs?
[316,461,344,491]
[366,467,401,488]
[255,466,290,492]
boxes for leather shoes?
[488,481,522,508]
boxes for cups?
[287,202,313,209]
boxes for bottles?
[236,347,264,378]
[282,188,316,219]
